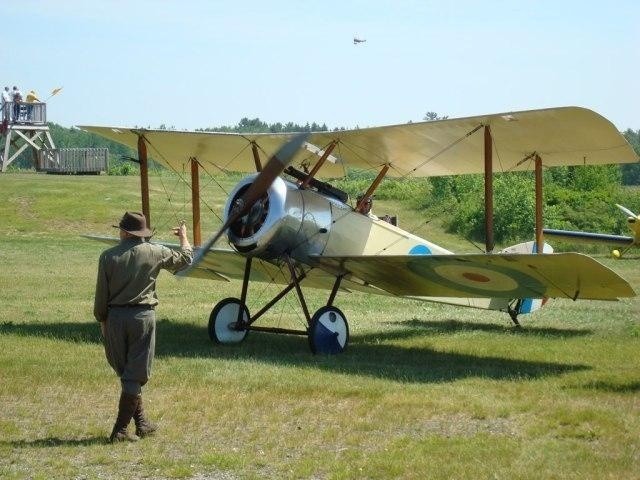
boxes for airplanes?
[74,105,640,355]
[532,202,640,259]
[352,33,367,46]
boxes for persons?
[93,210,195,443]
[25,90,41,121]
[10,85,23,125]
[12,91,24,124]
[355,190,379,221]
[1,86,14,126]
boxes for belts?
[106,303,157,312]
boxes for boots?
[132,393,161,439]
[106,391,140,444]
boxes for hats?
[111,210,154,239]
